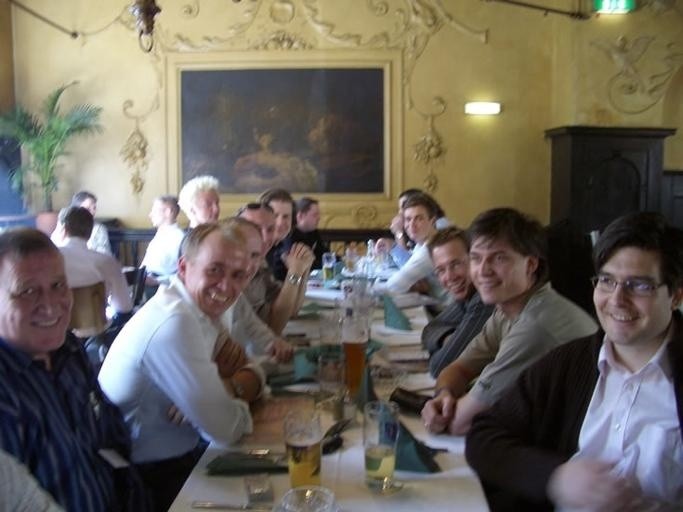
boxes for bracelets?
[229,375,246,398]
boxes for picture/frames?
[168,50,404,212]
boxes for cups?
[280,246,399,512]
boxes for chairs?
[125,267,144,304]
[70,281,113,340]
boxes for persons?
[51,206,135,347]
[232,105,353,195]
[1,448,68,512]
[369,187,457,317]
[464,210,683,511]
[136,174,341,363]
[96,222,266,507]
[420,224,494,379]
[0,227,150,512]
[420,205,600,438]
[50,189,111,254]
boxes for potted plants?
[0,80,106,239]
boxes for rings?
[423,421,428,429]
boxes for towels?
[263,336,384,415]
[381,293,413,330]
[376,401,425,447]
[300,302,335,310]
[379,414,442,474]
[288,312,321,321]
[205,449,288,478]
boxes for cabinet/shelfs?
[542,122,676,243]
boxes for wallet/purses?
[206,450,291,475]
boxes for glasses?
[589,273,668,297]
[235,202,275,223]
